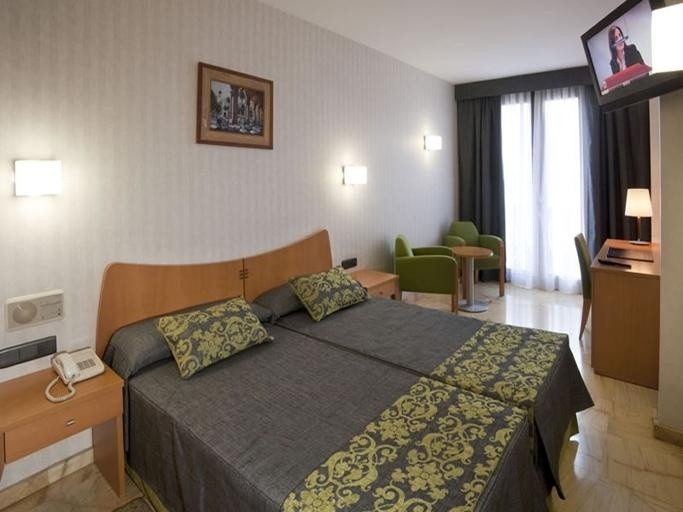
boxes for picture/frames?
[196,61,273,150]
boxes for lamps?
[625,188,652,245]
[15,160,62,196]
[344,165,367,184]
[425,135,443,151]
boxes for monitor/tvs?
[580,0,683,113]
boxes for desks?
[452,246,494,312]
[589,238,659,391]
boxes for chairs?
[574,233,592,338]
[445,221,504,296]
[394,234,459,314]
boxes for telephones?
[51,346,105,385]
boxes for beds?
[96,258,551,512]
[245,228,595,502]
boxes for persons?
[604,26,648,84]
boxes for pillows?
[102,264,372,381]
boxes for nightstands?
[349,269,400,301]
[0,362,125,498]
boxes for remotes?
[598,259,631,268]
